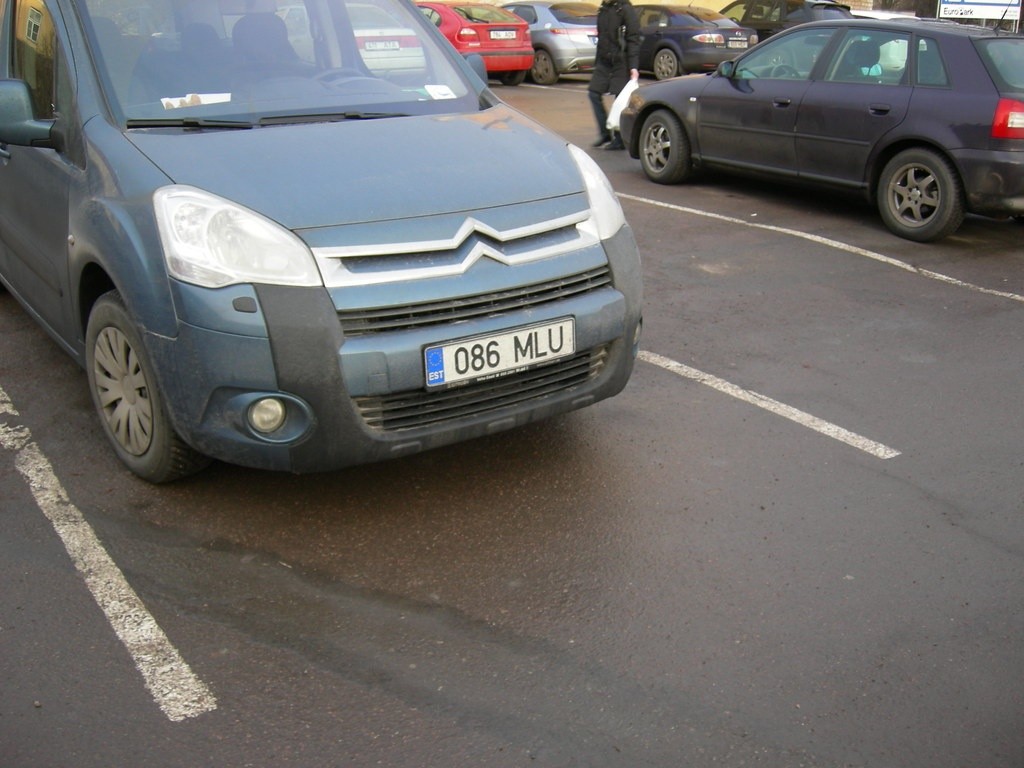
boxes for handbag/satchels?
[605,78,639,130]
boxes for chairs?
[226,13,328,92]
[647,14,659,25]
[900,51,946,87]
[829,41,880,83]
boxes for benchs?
[92,14,225,108]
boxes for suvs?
[617,15,1024,242]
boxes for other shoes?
[604,138,626,150]
[592,132,611,147]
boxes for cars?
[221,0,427,87]
[480,0,603,87]
[0,0,647,486]
[631,3,759,81]
[717,0,856,55]
[399,0,535,87]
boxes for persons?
[232,13,357,82]
[586,0,641,150]
